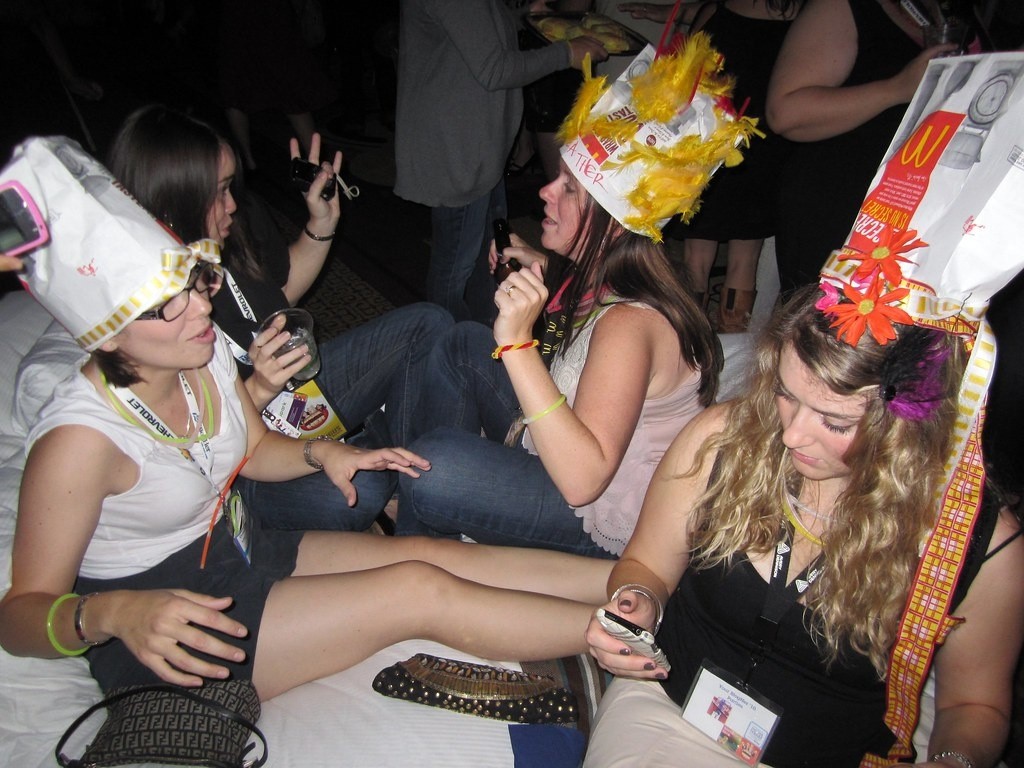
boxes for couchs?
[0,289,601,768]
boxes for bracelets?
[304,224,336,242]
[522,394,567,425]
[491,339,540,361]
[929,751,975,768]
[46,593,92,657]
[74,591,109,646]
[610,583,663,636]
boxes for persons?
[582,275,1024,768]
[0,255,22,271]
[0,226,616,702]
[1,0,1024,327]
[389,141,725,561]
[109,103,455,532]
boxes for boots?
[693,292,710,312]
[717,286,755,334]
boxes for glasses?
[137,261,214,321]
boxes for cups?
[257,308,320,380]
[921,24,969,61]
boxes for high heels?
[506,152,536,177]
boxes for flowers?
[821,221,925,349]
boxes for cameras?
[288,156,337,202]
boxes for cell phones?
[597,609,671,673]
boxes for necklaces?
[571,286,617,328]
[776,485,849,549]
[92,352,214,451]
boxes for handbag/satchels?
[55,679,268,768]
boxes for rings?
[643,5,647,12]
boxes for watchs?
[303,435,333,469]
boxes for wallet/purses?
[372,651,580,725]
